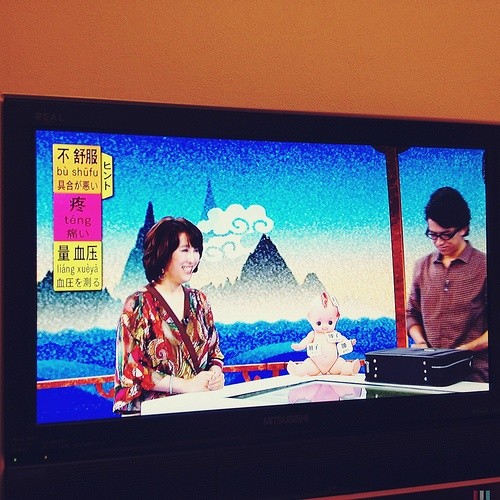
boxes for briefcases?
[364,348,473,387]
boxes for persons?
[112,215,226,415]
[286,291,361,376]
[404,185,491,384]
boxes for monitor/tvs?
[0,93,499,495]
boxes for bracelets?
[169,374,174,395]
[419,341,427,344]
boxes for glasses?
[424,227,460,240]
